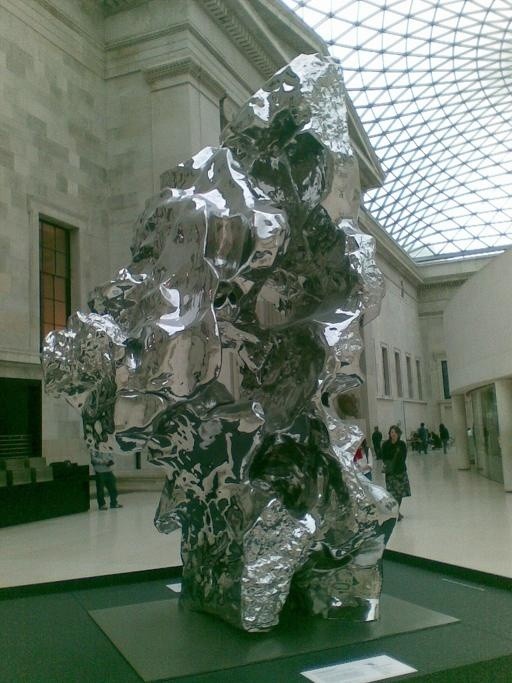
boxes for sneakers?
[100,503,108,510]
[115,503,124,508]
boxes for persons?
[352,437,373,481]
[372,423,449,460]
[380,424,411,521]
[91,453,123,510]
